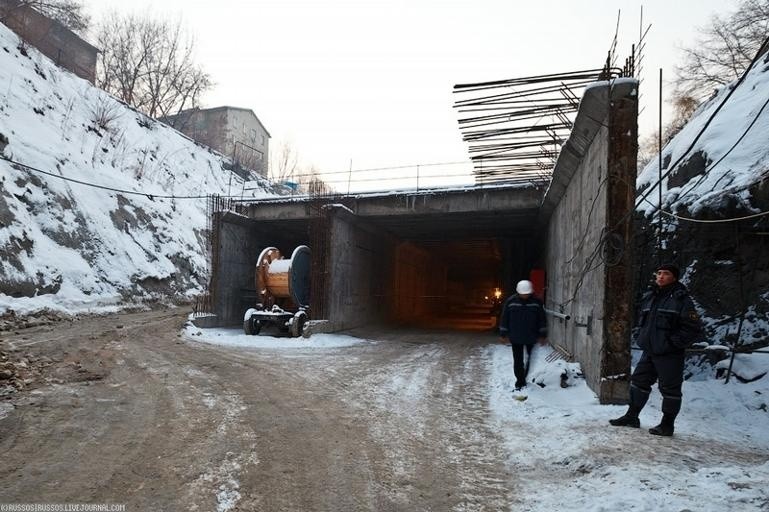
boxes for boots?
[608,385,651,428]
[648,395,682,437]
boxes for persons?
[608,262,699,438]
[496,276,550,393]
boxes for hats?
[657,262,681,281]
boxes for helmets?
[516,280,534,295]
